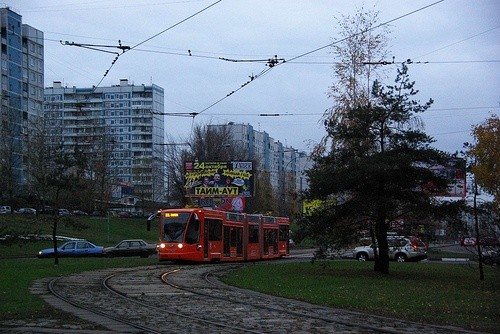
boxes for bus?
[146,208,291,265]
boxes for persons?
[200,173,234,189]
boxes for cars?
[0,204,162,218]
[103,240,158,258]
[0,233,85,245]
[464,238,476,246]
[36,241,104,257]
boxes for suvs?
[351,235,428,263]
